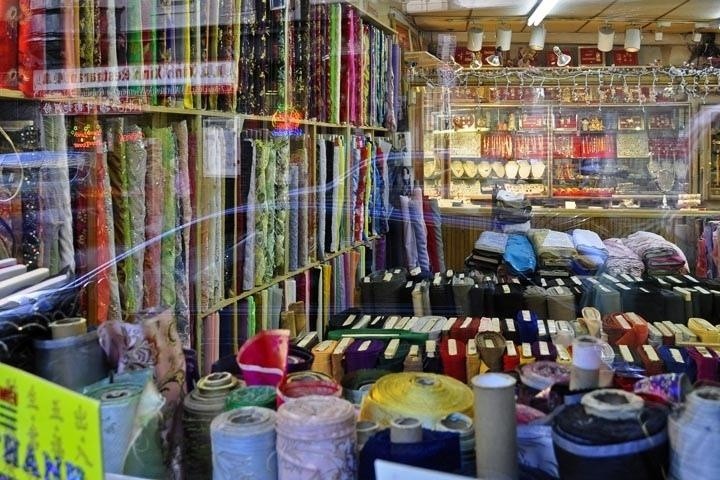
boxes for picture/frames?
[577,45,606,67]
[611,48,640,66]
[477,44,503,68]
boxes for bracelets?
[554,186,580,194]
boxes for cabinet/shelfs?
[0,0,406,382]
[422,100,720,203]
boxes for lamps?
[486,50,501,66]
[552,46,571,66]
[640,20,702,42]
[446,18,661,53]
[446,55,462,72]
[470,53,482,70]
[526,0,559,27]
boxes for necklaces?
[452,134,649,180]
